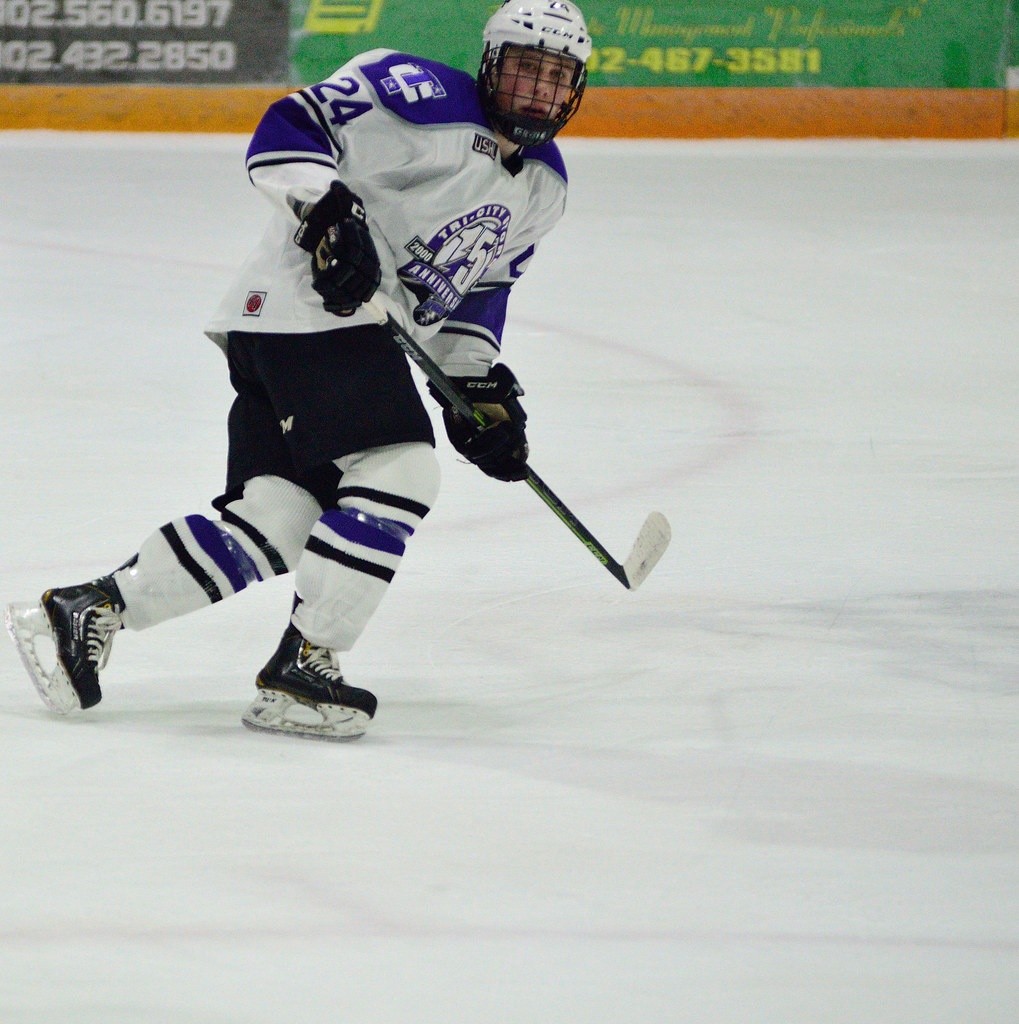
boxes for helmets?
[481,0,592,149]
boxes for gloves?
[293,179,383,313]
[426,364,529,482]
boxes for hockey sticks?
[365,296,672,593]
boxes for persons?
[7,0,592,742]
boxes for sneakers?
[6,577,122,709]
[241,624,376,737]
